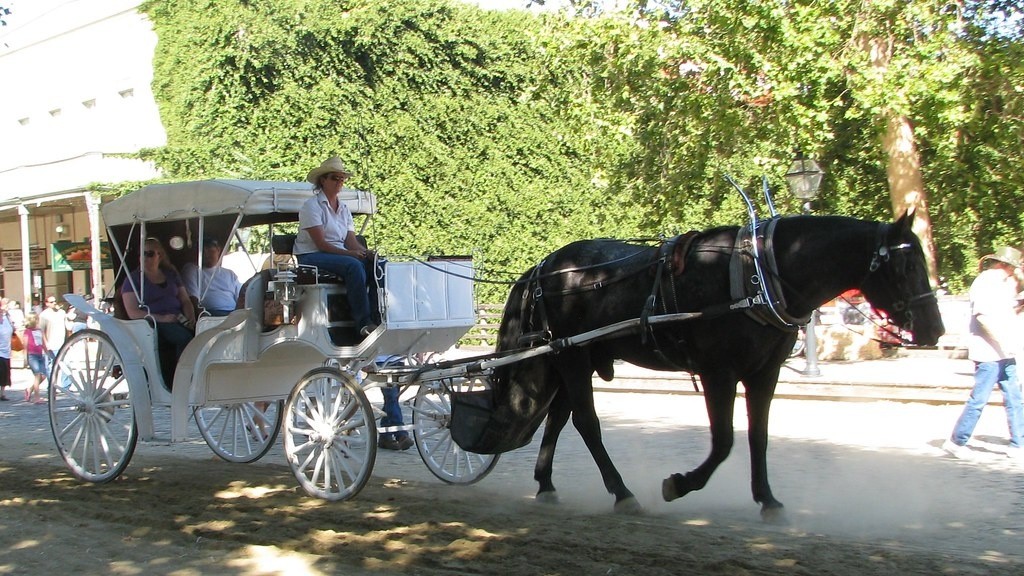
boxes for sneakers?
[399,435,414,451]
[378,440,401,450]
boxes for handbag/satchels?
[11,333,24,352]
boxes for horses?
[490,207,946,525]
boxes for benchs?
[236,270,316,326]
[113,286,128,320]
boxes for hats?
[306,157,354,185]
[978,246,1023,273]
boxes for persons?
[121,237,242,359]
[943,246,1024,461]
[295,157,388,336]
[372,354,415,451]
[0,287,115,405]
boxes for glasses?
[47,300,56,303]
[204,233,218,250]
[327,175,344,181]
[144,250,154,257]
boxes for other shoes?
[246,424,259,441]
[31,398,45,404]
[360,324,377,340]
[261,432,269,441]
[24,388,31,401]
[61,390,73,393]
[1,394,10,401]
[943,438,976,460]
[1007,446,1024,458]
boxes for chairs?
[270,234,384,330]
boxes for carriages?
[48,180,946,525]
